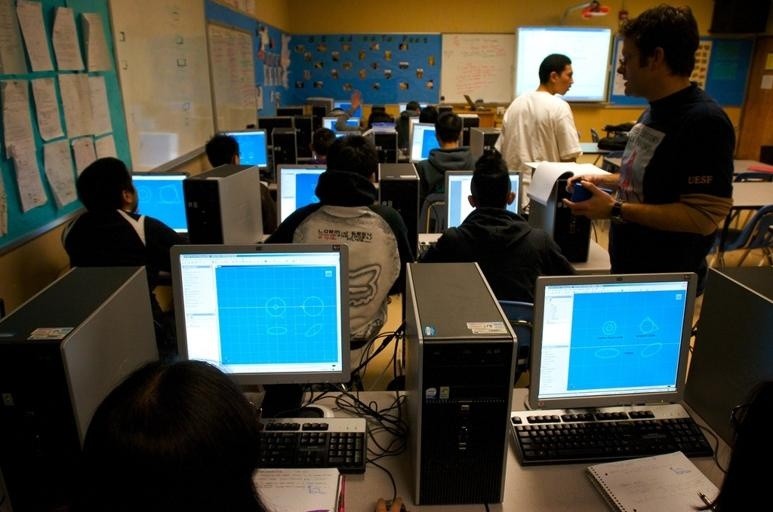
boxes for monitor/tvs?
[409,123,441,163]
[339,103,361,118]
[334,131,362,138]
[129,171,192,236]
[322,117,360,131]
[258,116,295,148]
[312,105,327,120]
[444,171,523,232]
[399,102,428,114]
[170,244,351,419]
[276,107,304,119]
[409,116,419,142]
[293,115,314,144]
[276,164,328,228]
[218,129,270,172]
[528,271,701,411]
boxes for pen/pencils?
[338,475,345,512]
[698,490,716,511]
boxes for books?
[584,451,722,511]
[250,465,343,512]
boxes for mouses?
[383,496,406,512]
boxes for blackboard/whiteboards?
[0,0,214,258]
[439,32,516,108]
[206,21,260,136]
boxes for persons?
[327,92,439,153]
[692,379,773,509]
[307,129,337,162]
[415,153,577,303]
[563,6,736,299]
[58,155,180,334]
[206,134,278,233]
[418,114,479,212]
[264,134,413,384]
[73,358,404,511]
[494,55,581,214]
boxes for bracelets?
[610,198,625,223]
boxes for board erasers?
[247,124,254,128]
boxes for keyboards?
[510,402,715,467]
[251,415,367,475]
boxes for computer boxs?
[403,261,518,506]
[0,265,160,511]
[683,264,773,450]
